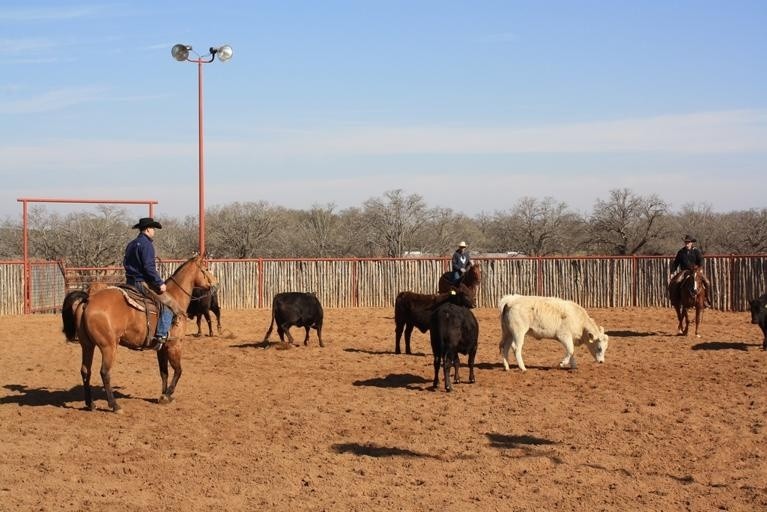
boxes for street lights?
[172,41,235,257]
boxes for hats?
[681,235,697,243]
[457,241,468,247]
[132,217,163,230]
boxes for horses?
[59,252,220,414]
[667,260,711,338]
[437,265,483,294]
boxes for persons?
[124,218,174,345]
[670,234,711,306]
[453,241,471,282]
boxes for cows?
[429,303,480,391]
[393,282,477,354]
[495,293,610,373]
[186,284,223,338]
[264,291,324,348]
[746,292,767,351]
